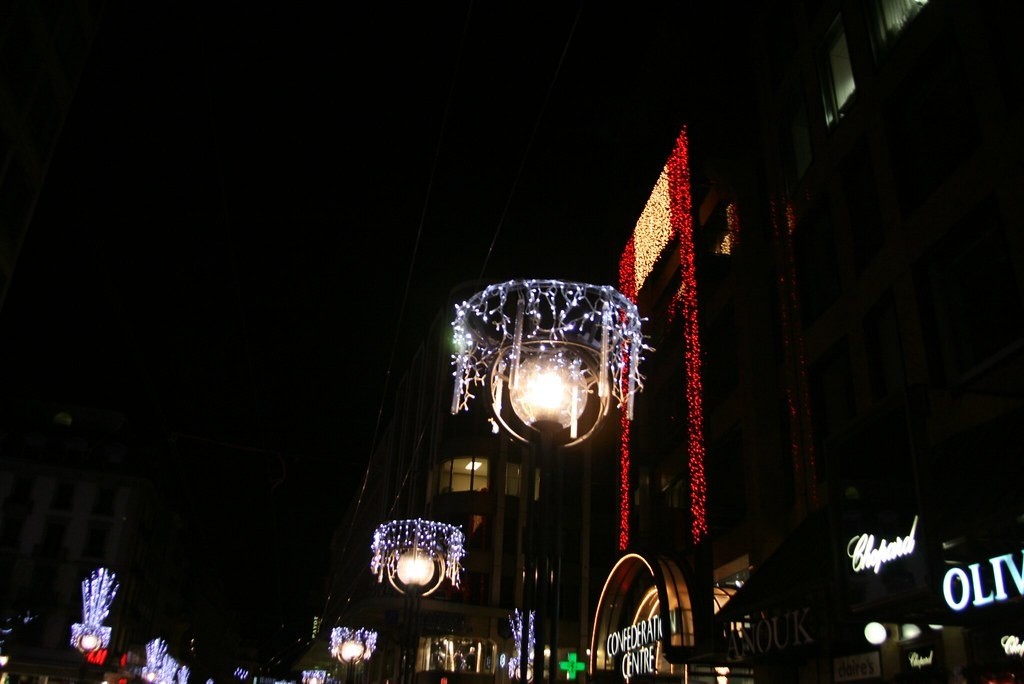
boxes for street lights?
[450,278,657,684]
[328,626,378,684]
[370,517,466,684]
